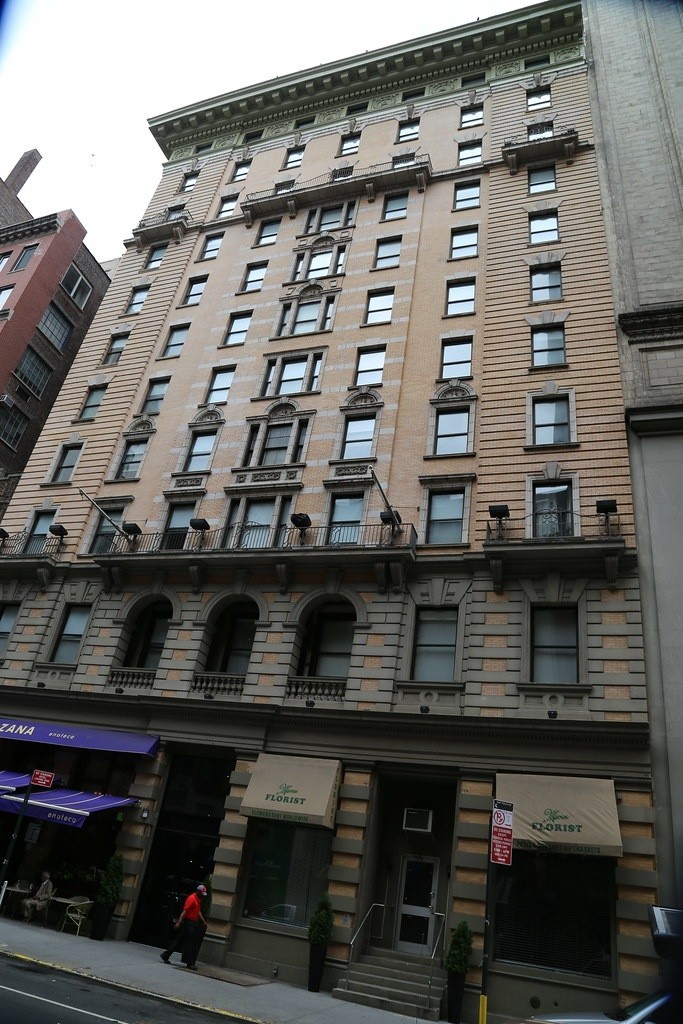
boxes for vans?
[524,903,683,1024]
[261,903,306,924]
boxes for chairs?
[2,881,95,936]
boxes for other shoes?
[22,917,28,922]
[160,951,170,964]
[187,963,197,970]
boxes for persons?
[160,884,207,970]
[21,872,53,921]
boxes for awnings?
[0,770,141,828]
[239,752,343,828]
[495,773,624,858]
[0,718,160,758]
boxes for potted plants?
[181,876,213,964]
[444,918,473,1024]
[306,890,334,992]
[90,850,124,940]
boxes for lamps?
[49,525,68,545]
[123,523,142,543]
[0,528,9,543]
[291,513,311,537]
[190,519,210,539]
[489,505,510,525]
[596,500,617,522]
[37,682,558,719]
[380,511,402,534]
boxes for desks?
[1,886,30,918]
[49,897,77,931]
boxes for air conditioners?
[402,808,433,833]
[0,395,14,409]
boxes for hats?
[197,885,207,896]
